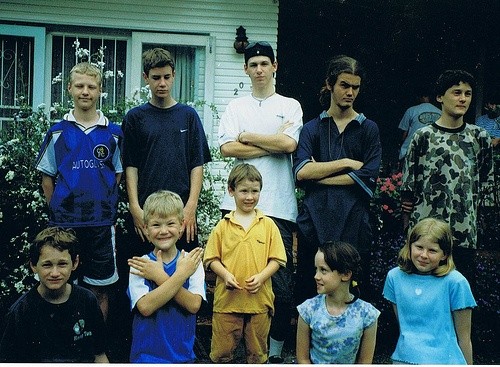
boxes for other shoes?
[267,355,284,364]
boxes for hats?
[244,41,275,78]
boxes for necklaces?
[329,107,354,161]
[251,92,276,107]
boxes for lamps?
[234,26,249,55]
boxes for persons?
[296,240,382,364]
[400,69,496,363]
[118,48,212,259]
[0,226,110,364]
[35,62,126,325]
[128,190,210,364]
[218,40,299,364]
[398,85,442,172]
[283,55,382,353]
[482,94,500,150]
[382,218,477,365]
[202,163,288,364]
